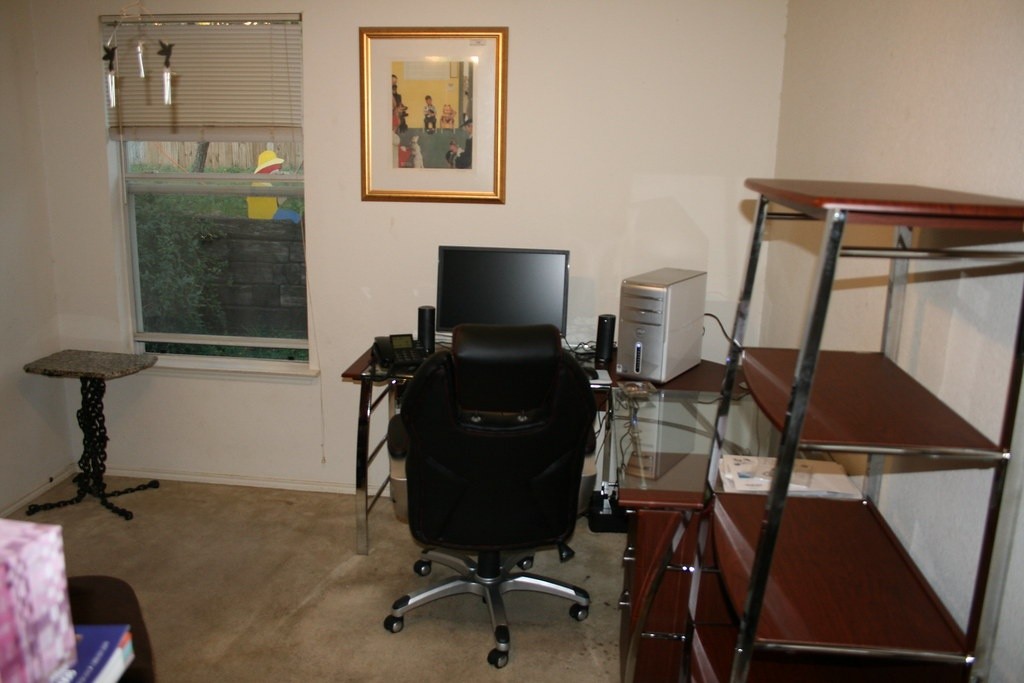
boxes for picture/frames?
[360,26,509,203]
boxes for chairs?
[384,324,597,669]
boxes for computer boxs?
[616,268,707,383]
[623,388,699,479]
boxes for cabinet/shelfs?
[608,387,863,683]
[677,179,1024,683]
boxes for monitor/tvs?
[438,246,569,337]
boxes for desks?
[21,349,160,520]
[339,341,751,557]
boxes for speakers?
[418,305,435,354]
[595,314,616,365]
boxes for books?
[53,625,134,683]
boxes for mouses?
[582,366,599,380]
